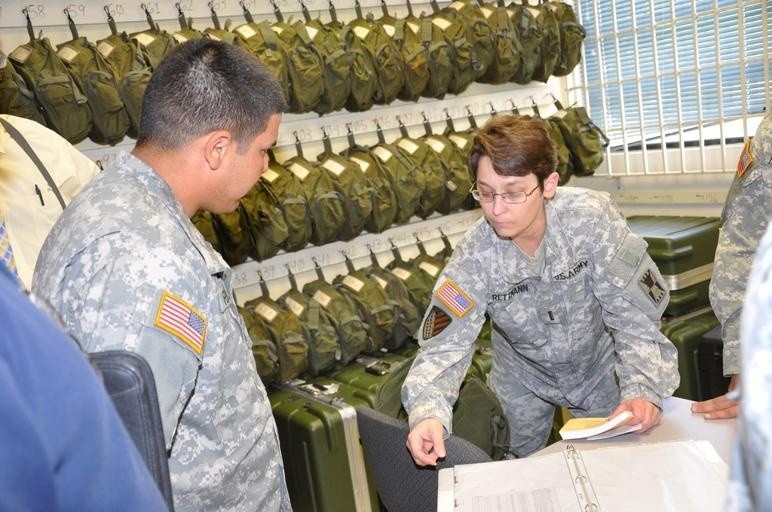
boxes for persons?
[1,112,100,304]
[26,40,295,511]
[403,114,679,469]
[1,263,171,510]
[689,112,770,419]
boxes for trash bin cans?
[701,325,730,399]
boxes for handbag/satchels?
[236,227,460,389]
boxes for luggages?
[627,214,730,402]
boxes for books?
[558,410,634,441]
[432,438,732,511]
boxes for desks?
[524,396,738,511]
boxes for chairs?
[356,402,495,511]
